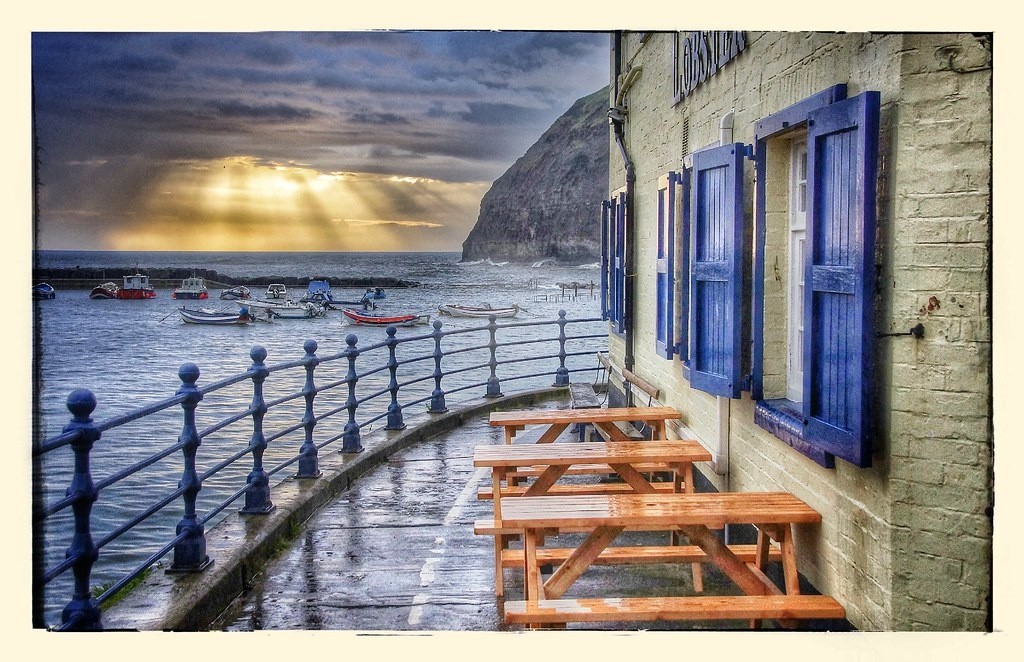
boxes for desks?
[498,489,823,601]
[489,405,683,445]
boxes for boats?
[118,273,156,301]
[362,292,387,300]
[265,283,287,301]
[178,305,252,325]
[221,285,252,300]
[342,308,432,327]
[88,281,118,299]
[301,280,333,306]
[235,298,323,318]
[171,268,208,300]
[33,281,55,301]
[438,303,521,319]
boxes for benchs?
[476,482,697,500]
[507,462,676,487]
[592,368,660,443]
[569,351,612,434]
[502,545,784,568]
[501,594,846,630]
[473,439,713,518]
[473,518,725,596]
